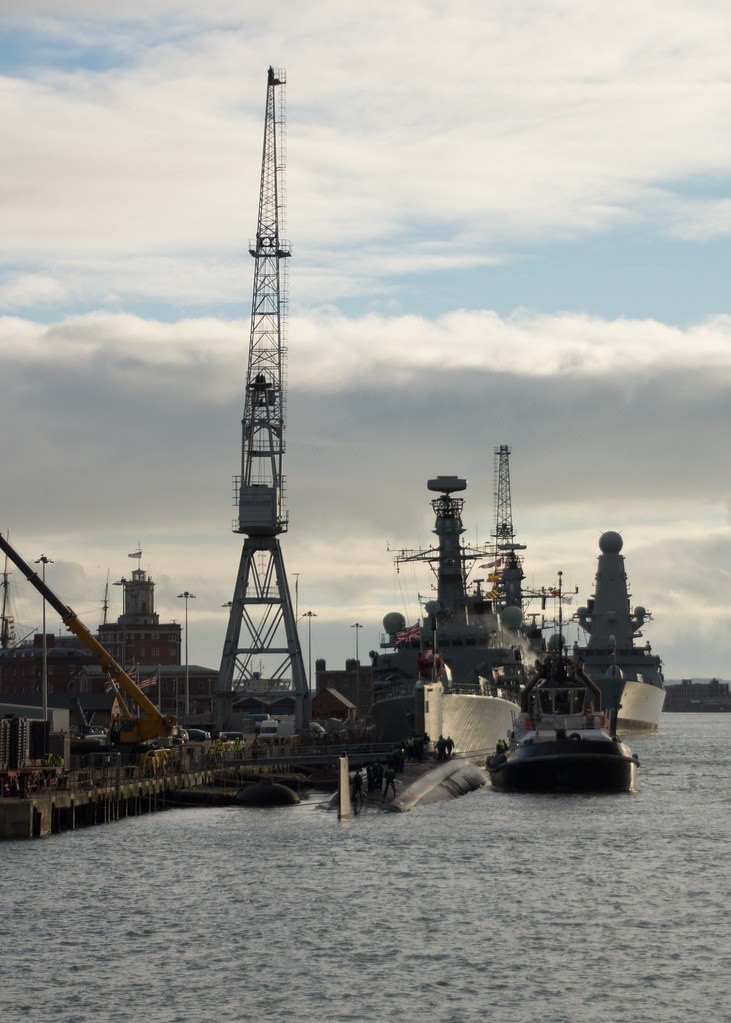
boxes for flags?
[562,597,573,605]
[103,666,136,694]
[128,551,142,559]
[481,585,500,600]
[479,558,502,569]
[551,589,561,598]
[394,623,420,644]
[137,669,157,690]
[486,571,501,583]
[415,648,441,665]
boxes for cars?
[70,712,376,746]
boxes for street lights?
[303,611,317,696]
[350,624,363,715]
[34,555,54,723]
[177,592,195,720]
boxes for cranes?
[208,67,314,733]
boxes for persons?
[383,764,396,797]
[156,746,169,766]
[373,758,384,772]
[366,764,375,793]
[446,737,454,760]
[202,731,446,773]
[496,738,505,753]
[54,753,65,768]
[501,739,508,749]
[375,761,384,793]
[42,753,53,768]
[351,771,363,803]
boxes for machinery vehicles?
[0,534,188,775]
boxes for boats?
[360,473,666,757]
[486,631,641,797]
[324,751,485,823]
